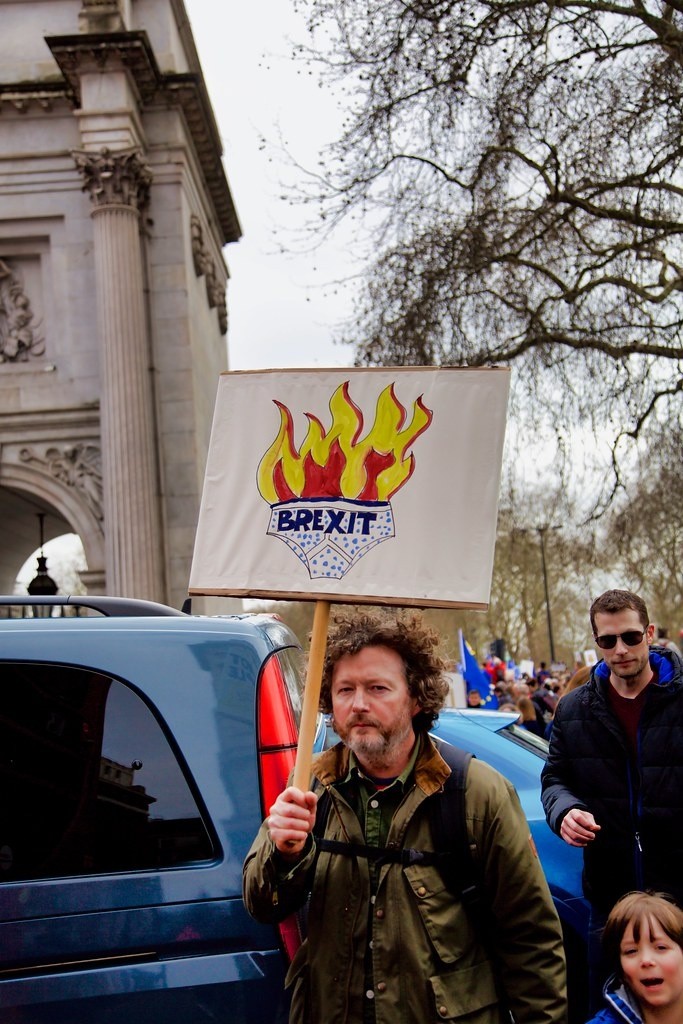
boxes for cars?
[315,710,610,1024]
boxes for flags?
[461,636,498,709]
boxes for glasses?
[595,630,646,649]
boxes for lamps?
[27,513,58,596]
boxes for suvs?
[0,594,306,1024]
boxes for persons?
[580,891,683,1024]
[468,661,592,741]
[540,590,683,996]
[654,628,682,660]
[243,610,568,1024]
